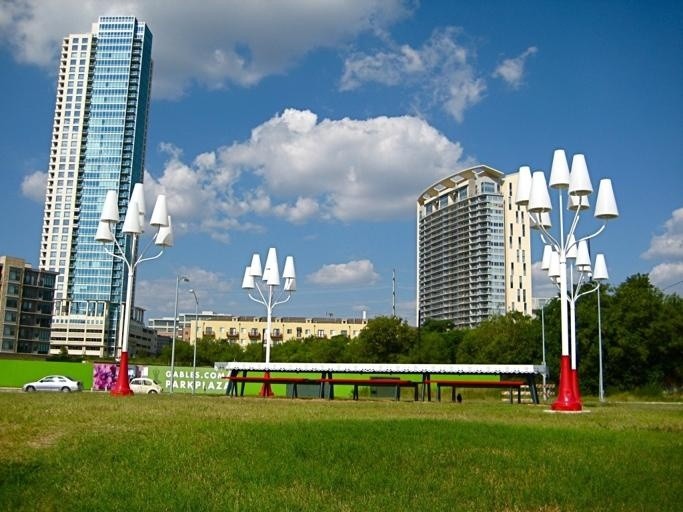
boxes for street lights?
[587,273,608,404]
[541,295,560,401]
[169,274,191,393]
[91,178,177,396]
[537,232,613,405]
[238,246,299,397]
[188,288,199,395]
[514,147,622,410]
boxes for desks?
[214,362,550,404]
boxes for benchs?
[222,377,528,404]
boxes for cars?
[20,374,85,393]
[128,376,163,395]
